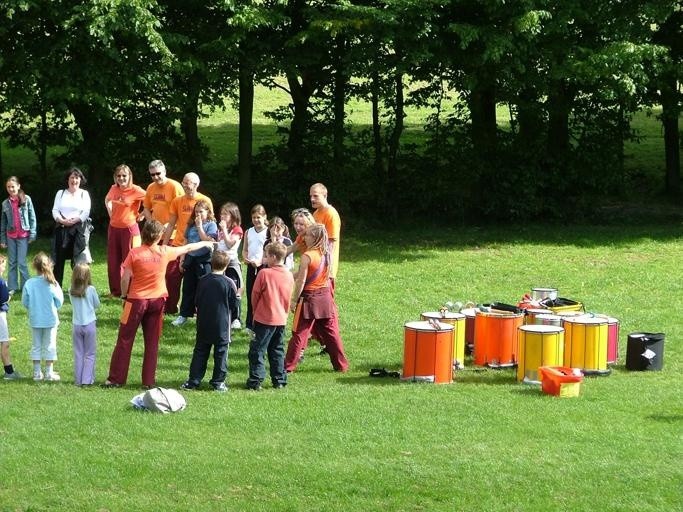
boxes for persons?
[263,216,295,271]
[245,240,296,390]
[169,200,218,326]
[67,262,100,385]
[240,203,272,334]
[163,170,213,314]
[0,254,25,380]
[215,201,242,330]
[282,225,348,373]
[181,252,239,391]
[308,183,343,298]
[284,207,338,357]
[19,251,64,381]
[0,175,38,293]
[142,158,185,314]
[50,168,91,289]
[104,163,149,298]
[102,219,220,388]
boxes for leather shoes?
[99,378,124,388]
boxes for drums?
[405,288,619,384]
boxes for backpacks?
[143,386,187,414]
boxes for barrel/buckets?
[517,286,619,383]
[403,320,454,384]
[473,306,523,368]
[461,300,490,352]
[420,311,466,369]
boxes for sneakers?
[3,372,26,381]
[179,382,201,390]
[231,319,242,330]
[31,370,61,381]
[248,379,285,391]
[170,315,187,327]
[298,345,328,360]
[208,385,228,393]
